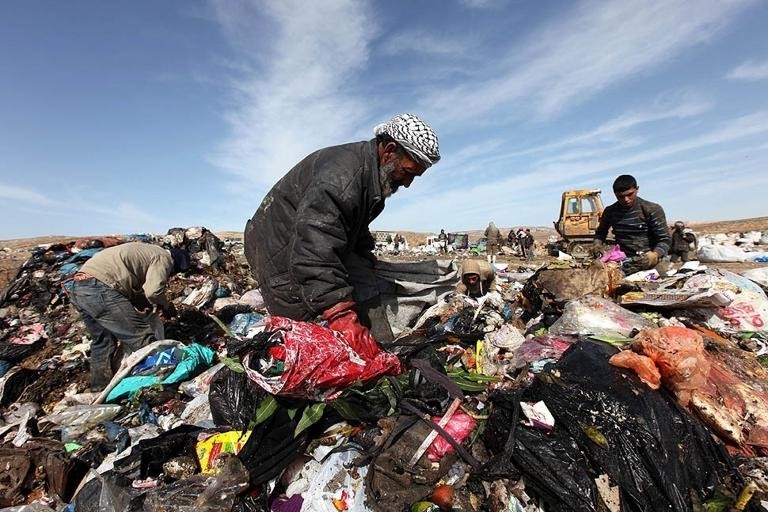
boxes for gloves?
[321,297,379,359]
[592,239,604,259]
[637,251,659,270]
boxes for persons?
[498,238,504,252]
[593,175,670,274]
[394,235,399,251]
[669,221,694,262]
[484,222,500,264]
[573,202,579,214]
[455,256,497,299]
[244,112,442,334]
[438,229,448,254]
[508,228,534,259]
[69,241,189,392]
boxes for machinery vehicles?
[553,189,617,260]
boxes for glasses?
[467,277,476,281]
